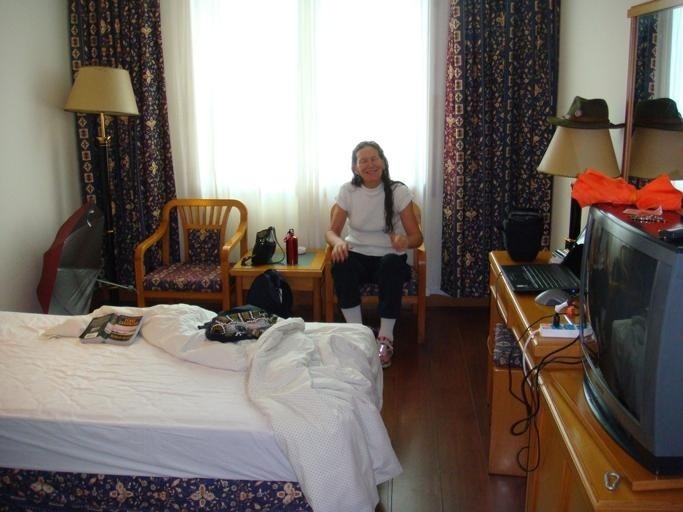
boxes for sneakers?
[375,335,395,370]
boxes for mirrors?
[620,1,682,192]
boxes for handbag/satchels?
[251,226,276,266]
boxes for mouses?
[535,288,569,305]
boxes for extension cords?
[538,322,590,337]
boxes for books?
[78,312,144,347]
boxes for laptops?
[501,224,587,294]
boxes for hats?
[633,98,683,131]
[546,95,626,130]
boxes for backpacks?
[247,269,292,318]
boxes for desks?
[228,242,324,331]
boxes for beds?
[0,306,404,512]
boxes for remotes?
[659,223,683,238]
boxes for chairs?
[130,196,249,314]
[322,199,428,347]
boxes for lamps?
[534,125,624,267]
[59,62,143,307]
[627,126,682,197]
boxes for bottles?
[286,229,298,266]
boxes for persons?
[324,139,424,370]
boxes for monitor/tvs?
[565,206,683,478]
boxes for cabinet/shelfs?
[475,245,681,511]
